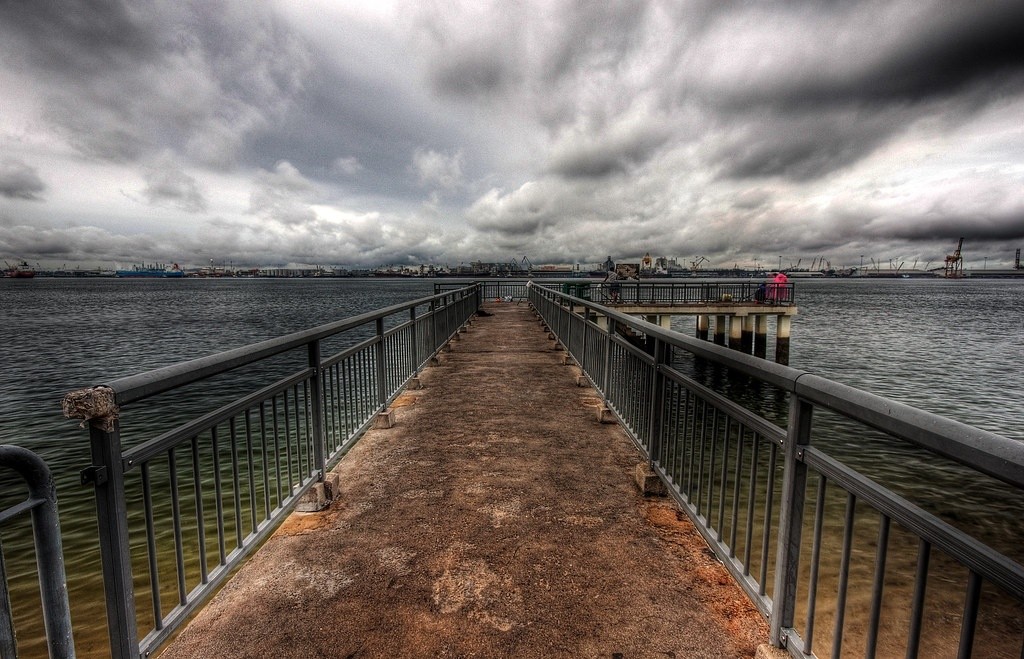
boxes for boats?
[4,260,35,278]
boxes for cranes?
[946,237,963,261]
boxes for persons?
[760,281,767,292]
[774,271,788,304]
[604,267,620,304]
